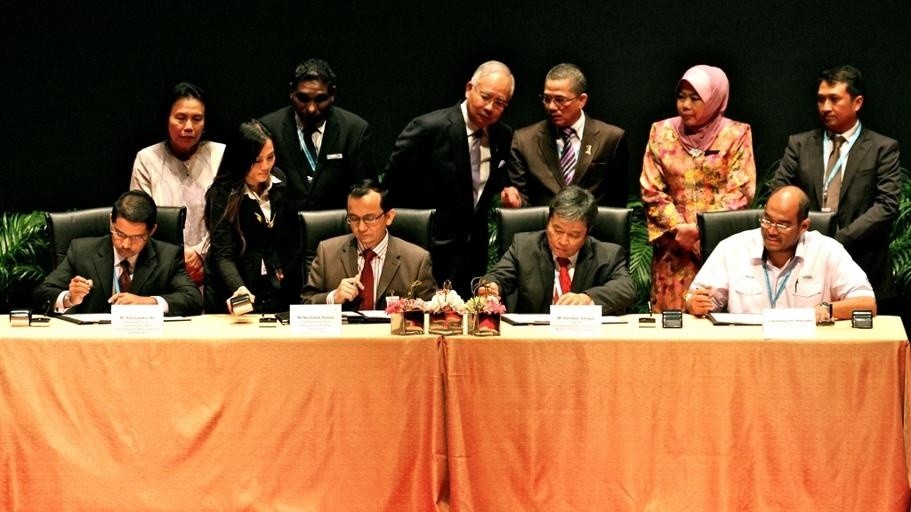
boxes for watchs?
[817,301,834,319]
[65,293,75,309]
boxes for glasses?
[758,212,801,234]
[479,90,509,112]
[538,94,578,106]
[110,220,150,242]
[344,211,387,225]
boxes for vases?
[391,313,502,336]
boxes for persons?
[498,61,629,210]
[31,190,203,316]
[128,79,228,275]
[378,59,516,298]
[684,182,879,326]
[302,176,437,311]
[476,184,637,316]
[637,61,757,312]
[769,62,902,293]
[202,114,305,315]
[257,56,375,211]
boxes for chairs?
[299,208,437,276]
[496,206,634,273]
[697,209,837,263]
[45,207,187,273]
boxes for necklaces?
[178,157,193,176]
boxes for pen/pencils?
[711,297,723,309]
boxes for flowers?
[384,294,507,316]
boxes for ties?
[118,261,132,293]
[301,126,319,176]
[555,257,573,303]
[559,128,576,186]
[821,136,847,213]
[469,128,484,210]
[355,249,376,310]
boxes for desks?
[444,316,911,512]
[0,314,444,512]
